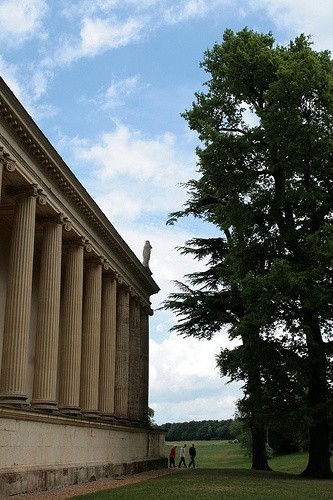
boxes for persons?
[178,444,187,468]
[168,445,178,468]
[188,443,196,468]
